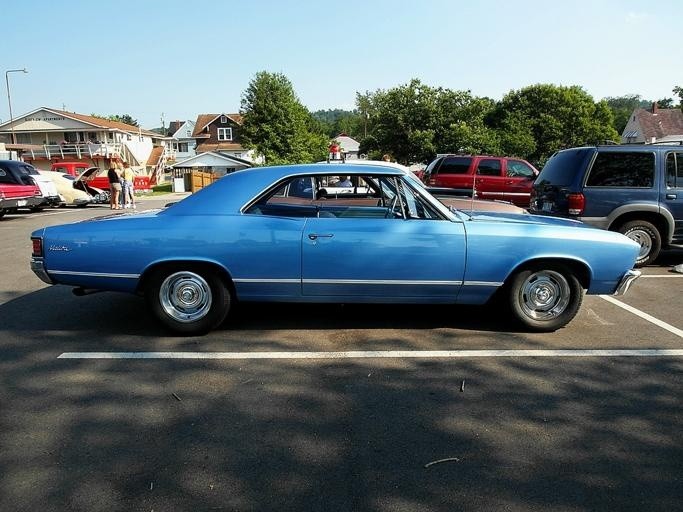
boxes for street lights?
[5,68,29,143]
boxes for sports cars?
[28,160,643,335]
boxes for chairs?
[246,205,342,219]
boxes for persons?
[330,175,353,188]
[107,161,136,210]
[382,154,390,162]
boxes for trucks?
[50,161,151,204]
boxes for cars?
[423,154,540,205]
[0,159,59,221]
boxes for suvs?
[530,138,683,268]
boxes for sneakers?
[111,204,136,210]
